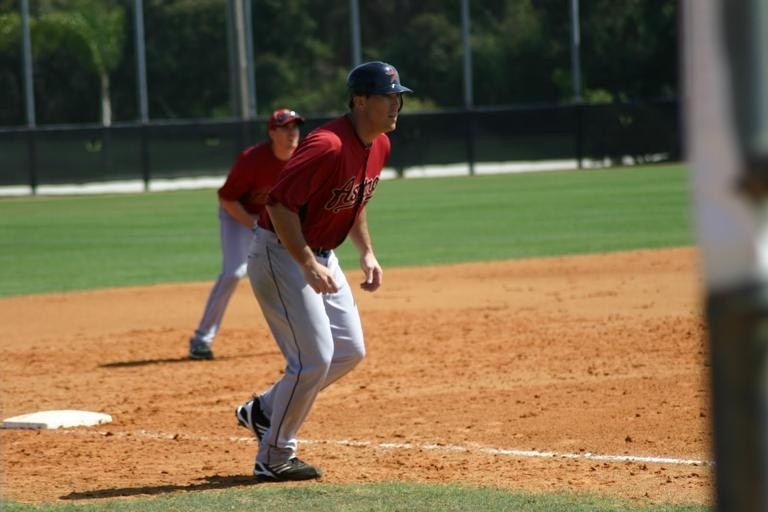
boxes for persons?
[235,60,414,480]
[189,108,305,361]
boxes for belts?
[316,248,331,258]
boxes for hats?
[346,61,415,95]
[268,108,306,130]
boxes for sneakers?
[235,397,270,444]
[189,339,213,359]
[253,457,317,481]
